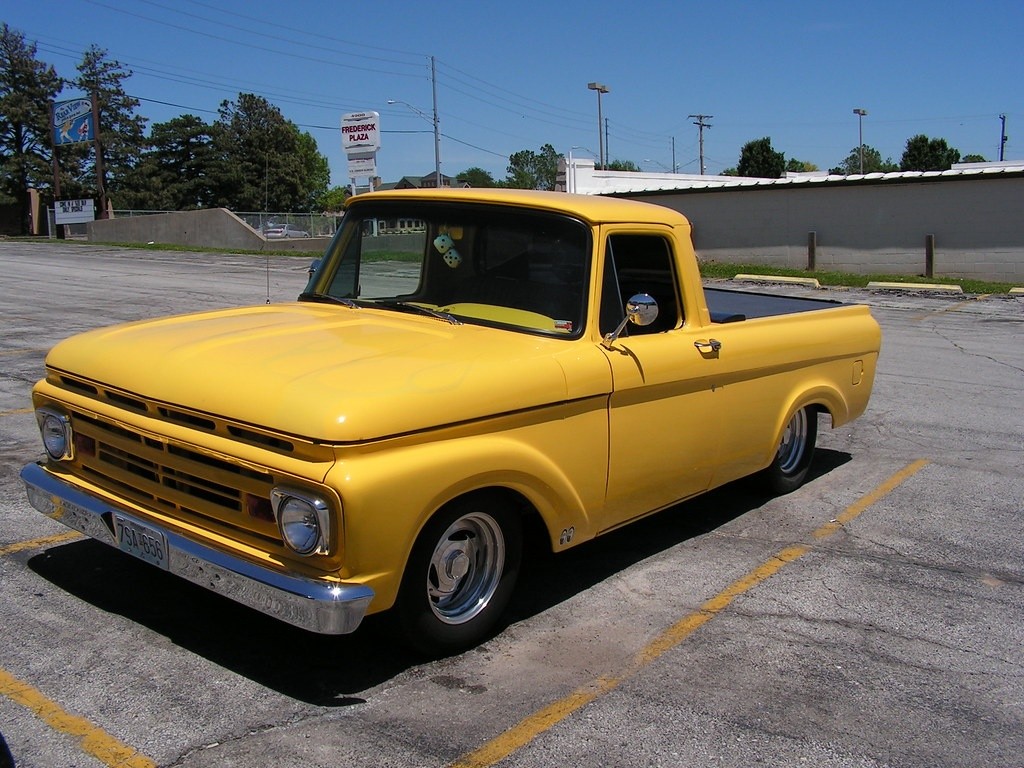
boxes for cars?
[263,223,312,239]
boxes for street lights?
[387,99,442,187]
[587,82,611,171]
[572,144,610,171]
[852,108,868,174]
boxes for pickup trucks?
[15,185,884,654]
